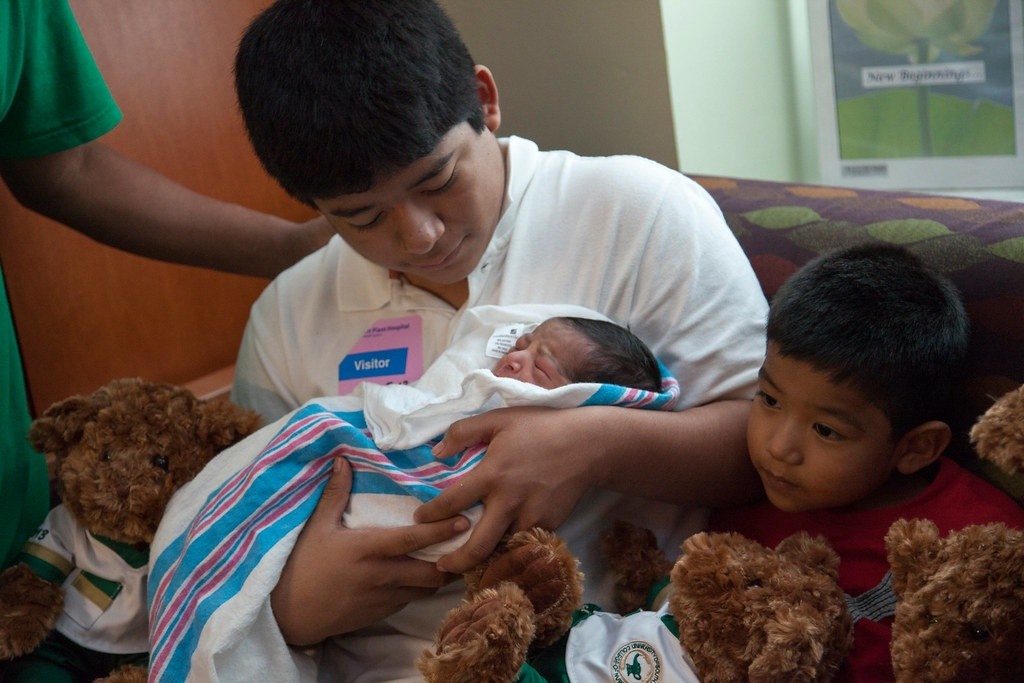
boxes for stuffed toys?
[418,383,1024,683]
[1,375,265,682]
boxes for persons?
[231,1,772,683]
[1,1,339,683]
[706,242,1023,682]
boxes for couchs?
[684,173,1023,462]
